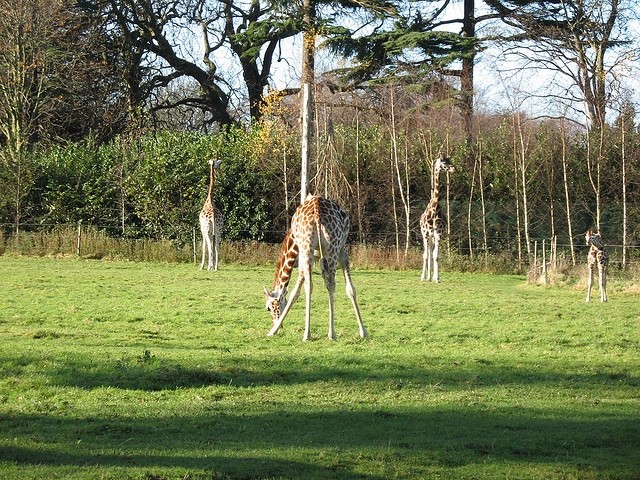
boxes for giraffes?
[419,150,458,283]
[583,226,611,305]
[260,193,372,343]
[200,157,225,271]
